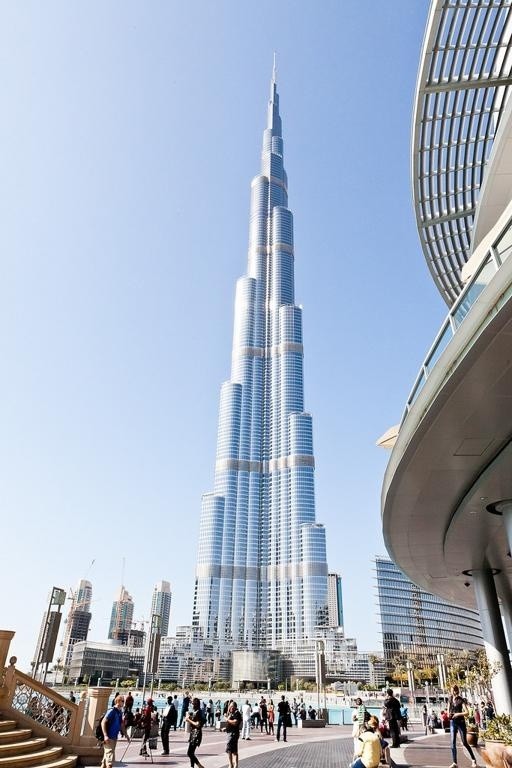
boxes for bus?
[400,694,450,703]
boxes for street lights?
[318,638,324,704]
[24,586,66,720]
[140,615,164,710]
[405,653,488,694]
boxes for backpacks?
[94,707,116,741]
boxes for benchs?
[126,726,144,738]
[383,734,408,745]
[297,718,327,729]
[216,720,244,731]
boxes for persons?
[138,699,154,757]
[446,686,477,768]
[351,688,408,767]
[99,696,131,768]
[223,702,242,768]
[161,696,176,755]
[112,693,317,741]
[26,690,76,732]
[186,698,203,767]
[423,699,494,735]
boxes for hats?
[147,698,155,703]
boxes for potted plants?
[466,723,479,746]
[483,713,508,768]
[497,715,512,768]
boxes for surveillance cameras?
[464,583,470,587]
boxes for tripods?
[120,717,153,764]
